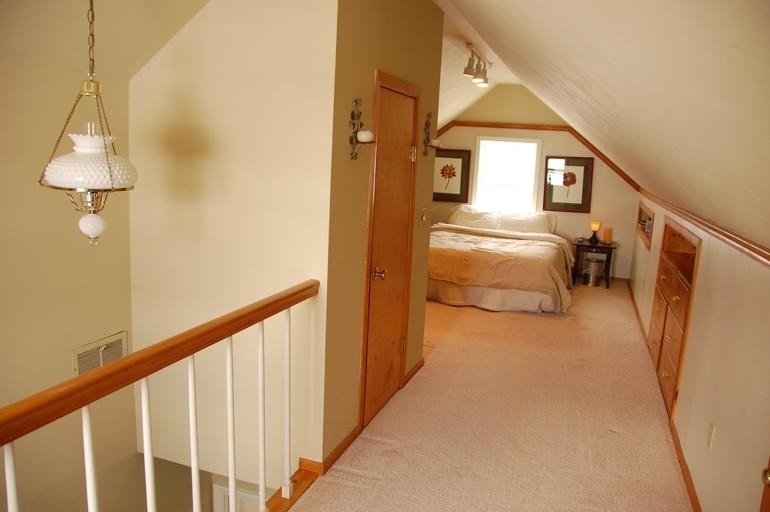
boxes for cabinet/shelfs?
[647,251,695,419]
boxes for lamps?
[463,43,493,88]
[347,96,376,161]
[38,0,136,246]
[423,112,440,157]
[588,221,602,244]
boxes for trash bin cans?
[583,258,604,287]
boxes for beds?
[426,205,576,314]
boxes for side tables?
[573,239,617,288]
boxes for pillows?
[498,214,556,234]
[449,205,497,229]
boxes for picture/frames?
[543,155,594,213]
[432,149,471,203]
